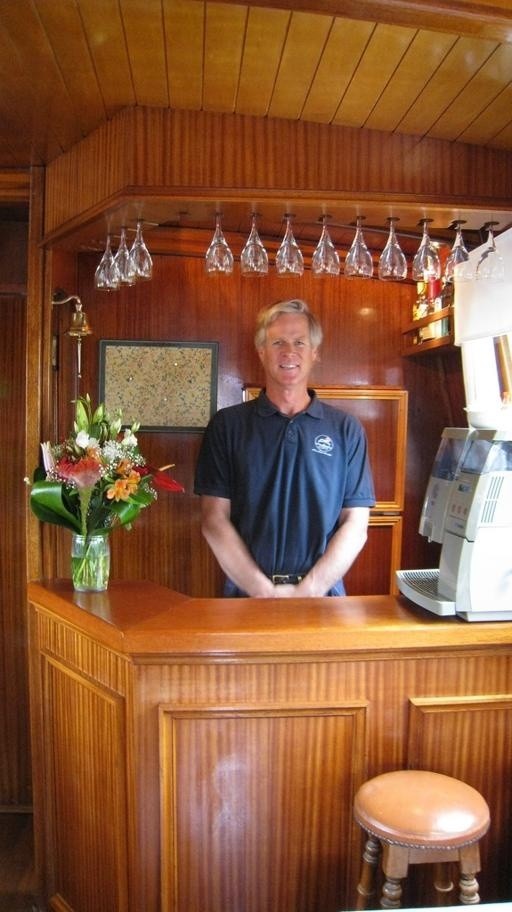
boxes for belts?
[265,574,303,585]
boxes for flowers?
[28,391,183,585]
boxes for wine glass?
[205,212,234,278]
[241,213,269,278]
[125,219,153,282]
[412,219,441,282]
[344,215,374,280]
[476,221,507,279]
[275,213,304,278]
[378,217,407,281]
[445,219,474,283]
[311,214,340,278]
[94,233,121,292]
[109,226,136,287]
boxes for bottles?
[412,281,429,344]
[442,282,454,336]
[434,276,446,338]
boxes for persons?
[192,299,375,597]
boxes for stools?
[353,767,494,908]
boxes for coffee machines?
[395,424,512,622]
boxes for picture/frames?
[98,339,219,432]
